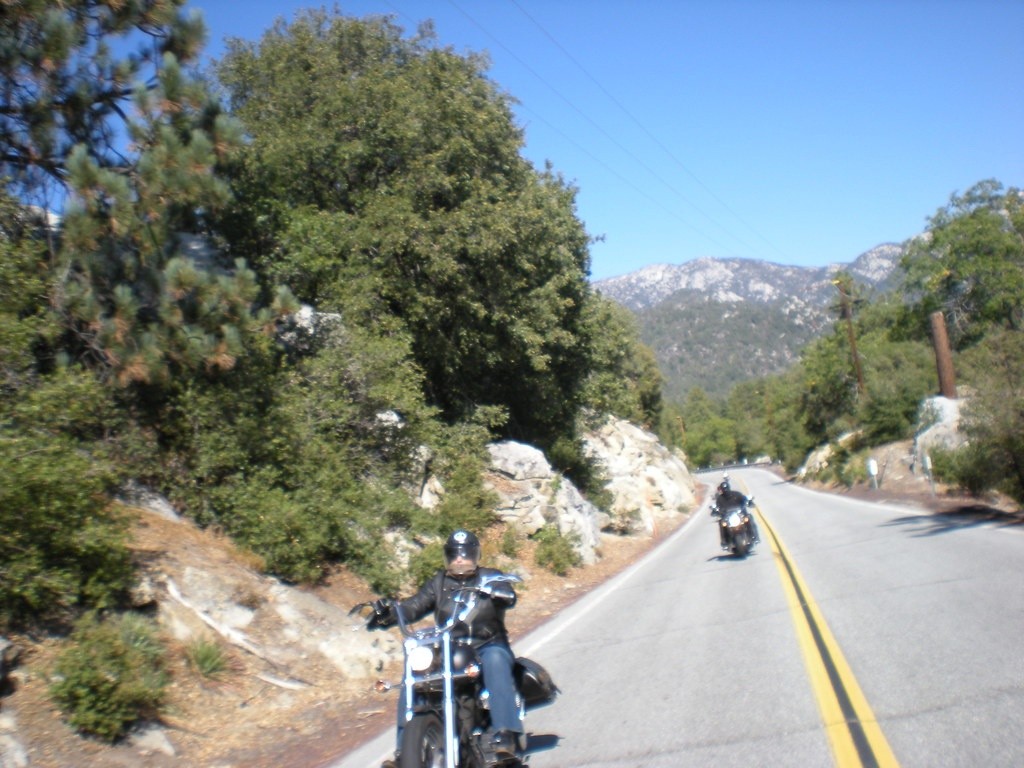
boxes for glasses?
[446,549,473,558]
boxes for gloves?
[489,583,517,608]
[345,601,379,632]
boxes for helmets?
[442,528,481,579]
[720,482,729,490]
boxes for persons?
[711,482,761,547]
[365,528,524,768]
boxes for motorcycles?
[708,496,760,558]
[347,574,560,768]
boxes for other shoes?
[381,760,399,768]
[495,731,517,755]
